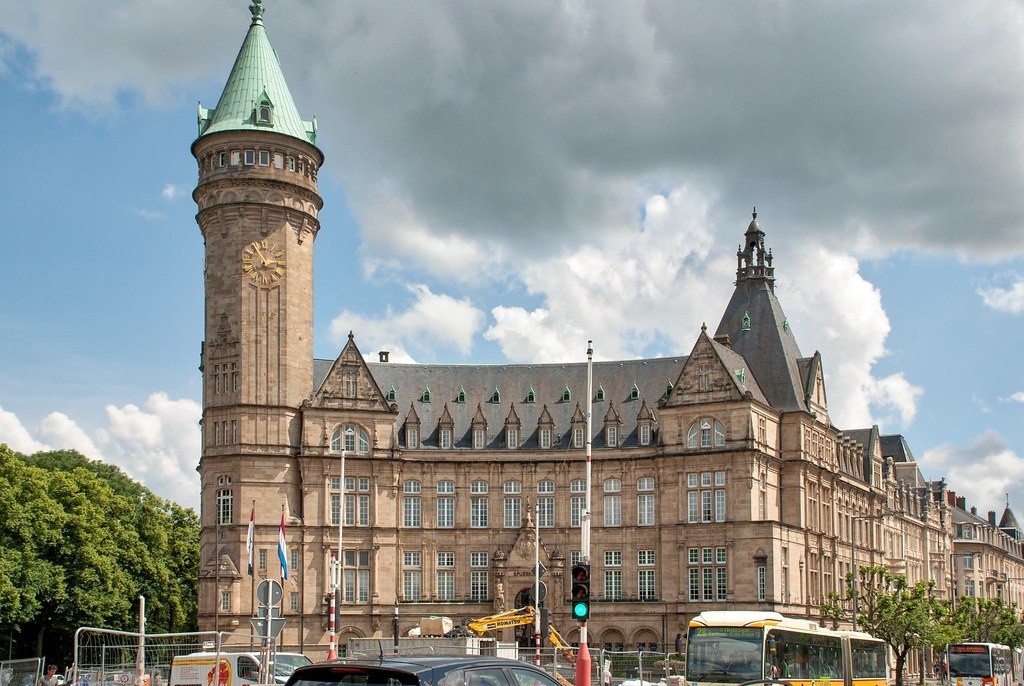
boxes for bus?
[685,611,891,686]
[943,643,1022,686]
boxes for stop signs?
[121,675,129,684]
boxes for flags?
[245,508,253,575]
[277,513,288,579]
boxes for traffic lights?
[571,564,591,619]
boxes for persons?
[722,652,860,677]
[38,664,58,686]
[749,653,767,672]
[67,670,89,686]
[933,659,948,681]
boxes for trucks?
[346,638,518,659]
[168,652,314,686]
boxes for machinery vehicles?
[420,605,576,680]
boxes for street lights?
[851,512,895,631]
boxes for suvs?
[285,655,563,686]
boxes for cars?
[52,675,65,686]
